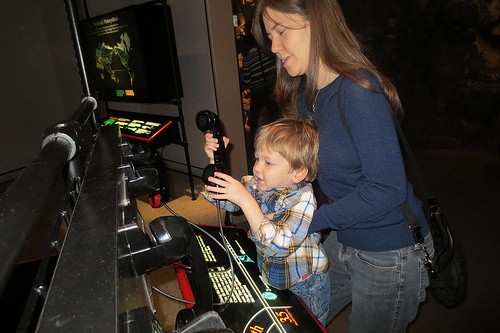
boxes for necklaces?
[309,69,326,113]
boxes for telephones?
[195,109,229,186]
[174,307,200,331]
[129,142,167,197]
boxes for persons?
[232,0,278,135]
[201,113,331,327]
[254,0,437,333]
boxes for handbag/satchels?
[423,200,468,307]
